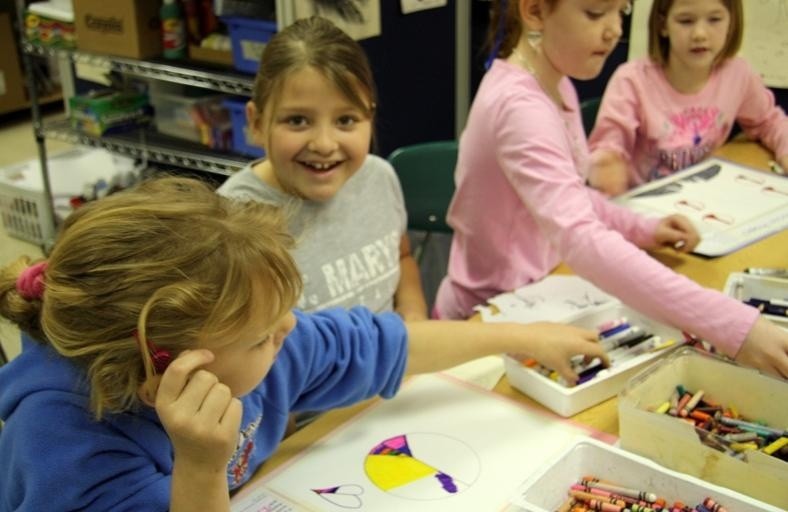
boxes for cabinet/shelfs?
[15,0,292,258]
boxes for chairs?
[388,136,470,265]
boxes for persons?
[212,16,428,324]
[0,172,610,512]
[587,2,788,201]
[432,1,788,379]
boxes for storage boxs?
[224,17,278,74]
[150,84,221,145]
[224,98,263,156]
[721,266,787,336]
[615,344,788,507]
[73,1,164,62]
[495,432,785,512]
[504,295,695,420]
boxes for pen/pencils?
[523,315,673,388]
[741,298,788,317]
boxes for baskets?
[0,146,135,246]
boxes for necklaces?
[509,46,556,104]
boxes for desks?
[220,138,788,512]
